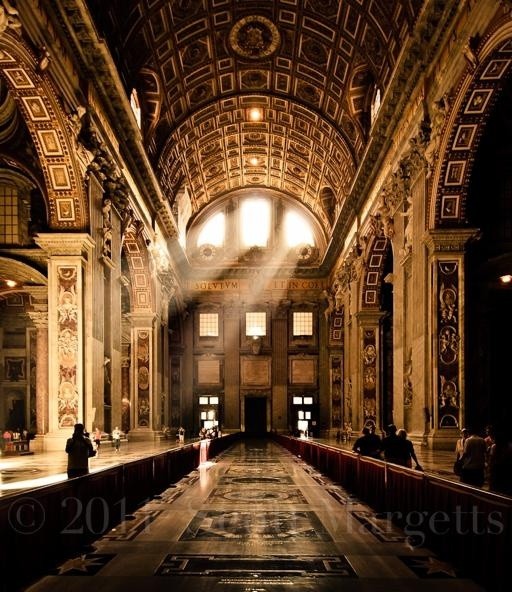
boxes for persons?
[178,426,186,443]
[22,426,29,450]
[64,423,97,476]
[396,429,424,472]
[112,426,121,452]
[13,429,20,452]
[454,424,495,488]
[380,423,406,466]
[368,424,380,438]
[199,427,205,439]
[4,428,13,450]
[95,427,101,449]
[352,425,383,461]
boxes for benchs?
[344,431,360,440]
[13,440,27,451]
[92,434,128,443]
[154,431,172,440]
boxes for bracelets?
[113,439,115,439]
